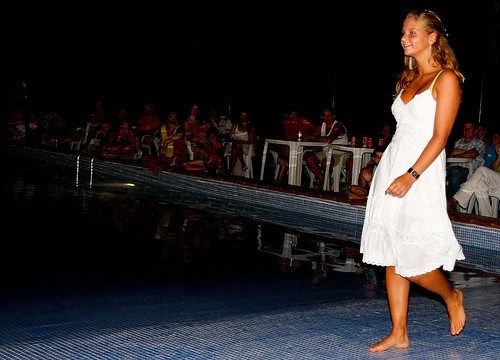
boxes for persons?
[224,110,257,176]
[302,108,350,190]
[275,108,318,189]
[482,132,500,169]
[444,121,487,212]
[473,122,492,150]
[377,123,394,150]
[446,144,500,218]
[358,7,467,353]
[346,161,378,201]
[0,100,234,181]
[362,146,385,186]
[102,196,386,293]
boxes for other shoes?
[447,198,456,208]
[242,165,247,173]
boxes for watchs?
[407,168,421,180]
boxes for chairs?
[70,125,162,156]
[222,141,255,178]
[269,149,348,192]
[456,158,500,218]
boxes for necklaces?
[242,122,248,124]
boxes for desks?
[257,221,369,276]
[322,143,376,192]
[259,139,331,191]
[445,157,479,216]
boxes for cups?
[296,130,303,141]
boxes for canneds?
[362,137,372,148]
[379,139,383,146]
[351,137,356,146]
